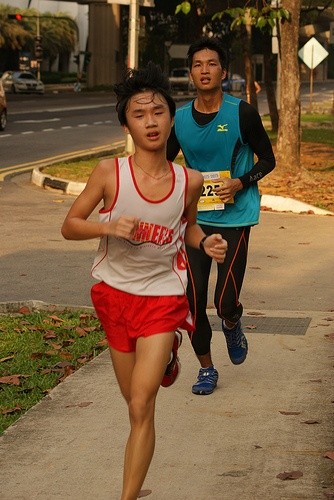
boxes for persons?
[60,73,230,500]
[170,41,278,394]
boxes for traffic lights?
[8,14,23,19]
[74,55,80,63]
[85,51,92,66]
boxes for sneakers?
[221,319,248,365]
[192,366,219,395]
[160,328,183,388]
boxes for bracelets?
[199,236,210,250]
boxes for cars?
[169,67,192,90]
[1,71,44,93]
[0,81,8,132]
[221,74,246,94]
